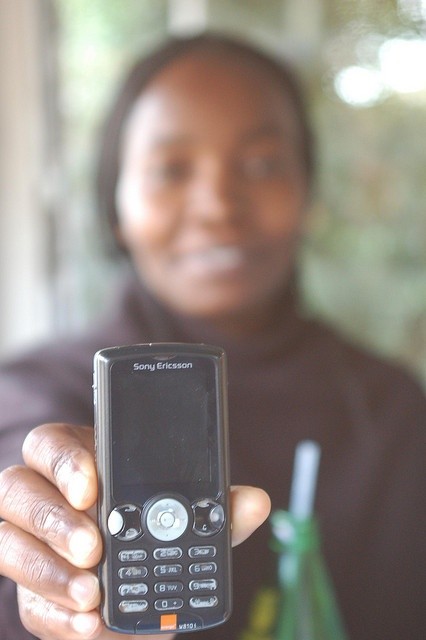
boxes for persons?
[1,29,426,640]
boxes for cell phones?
[92,343,233,634]
